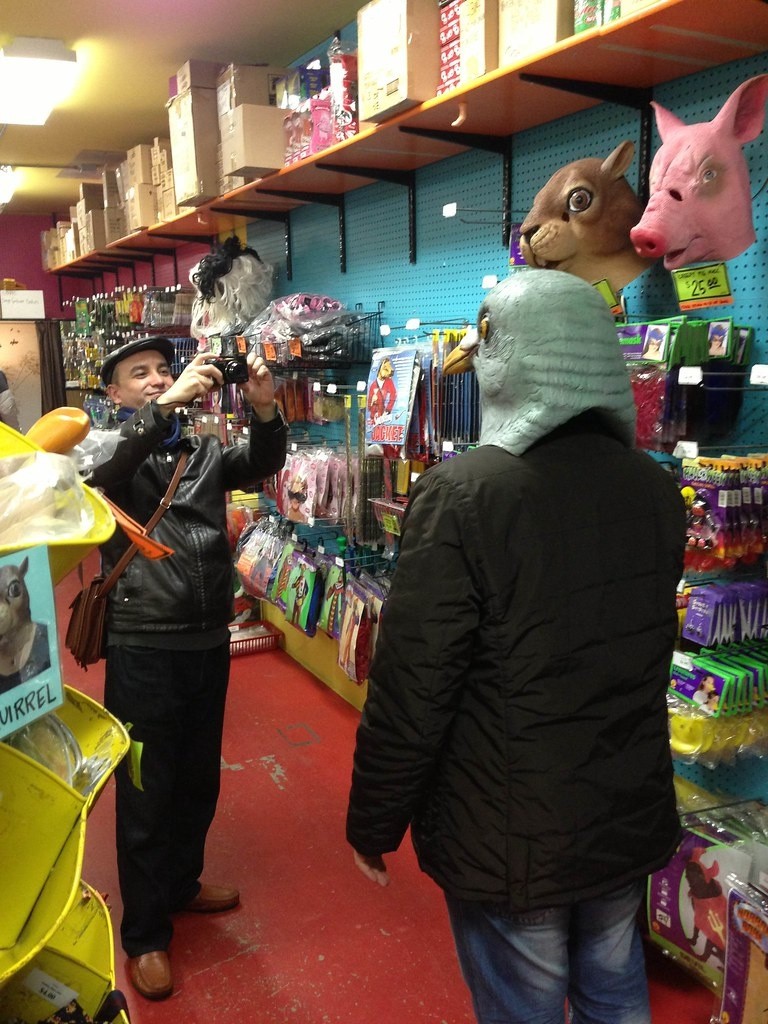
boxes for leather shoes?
[169,884,239,912]
[131,950,173,1001]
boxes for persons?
[80,331,292,1001]
[345,413,688,1024]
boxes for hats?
[100,336,176,388]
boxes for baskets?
[165,337,202,374]
[205,311,383,369]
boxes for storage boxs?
[39,0,664,271]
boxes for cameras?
[202,354,249,387]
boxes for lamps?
[0,36,78,126]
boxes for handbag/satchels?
[65,574,109,672]
